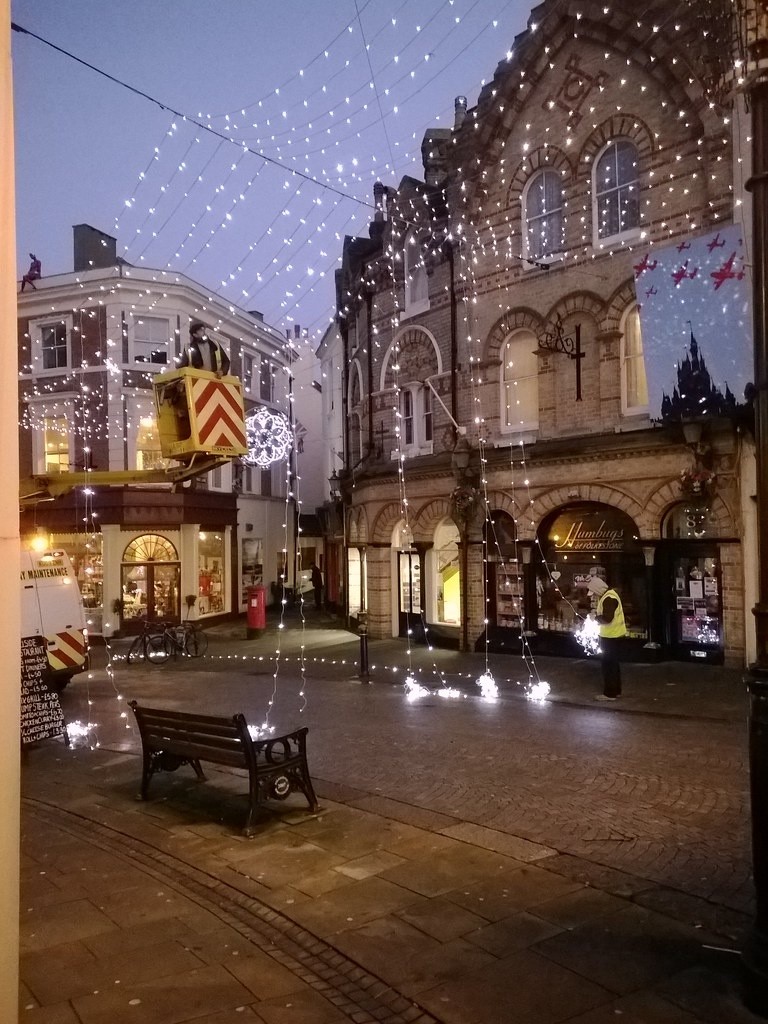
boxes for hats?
[587,590,594,596]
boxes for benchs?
[129,699,321,838]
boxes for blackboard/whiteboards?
[21,635,66,745]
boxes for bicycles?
[127,619,209,665]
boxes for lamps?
[451,439,481,492]
[683,413,705,465]
[327,472,353,507]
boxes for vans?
[21,548,91,693]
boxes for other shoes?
[616,695,620,697]
[595,694,615,702]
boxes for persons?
[175,324,230,379]
[308,561,322,609]
[586,576,627,701]
[17,252,41,294]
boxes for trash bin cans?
[281,588,294,609]
